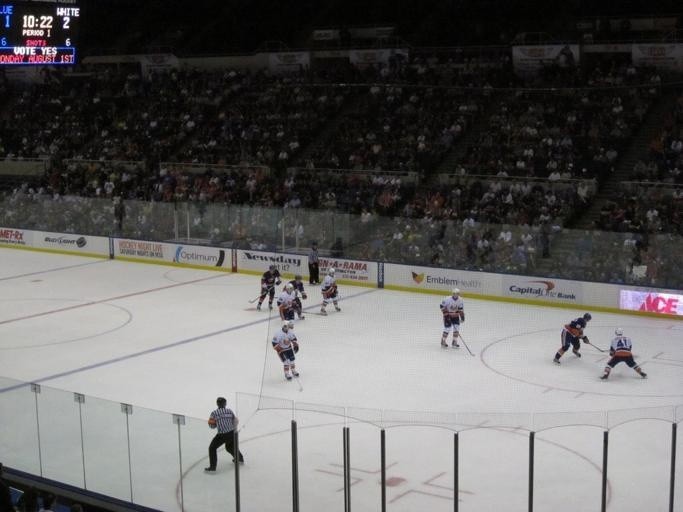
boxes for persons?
[276,283,301,328]
[0,25,683,289]
[0,463,84,512]
[282,274,307,318]
[553,312,591,363]
[271,323,300,380]
[204,397,244,472]
[598,327,647,380]
[256,265,281,309]
[320,267,340,313]
[440,288,465,348]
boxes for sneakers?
[600,374,608,379]
[441,340,460,347]
[269,304,273,308]
[205,468,216,472]
[297,313,305,318]
[640,372,647,377]
[232,456,244,462]
[335,306,340,311]
[321,308,326,312]
[286,370,299,379]
[257,304,261,309]
[553,358,560,363]
[573,350,581,357]
[310,280,321,284]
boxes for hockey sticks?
[589,343,610,353]
[451,317,478,356]
[248,285,276,304]
[282,353,303,393]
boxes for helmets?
[452,288,459,294]
[287,283,294,290]
[328,268,335,273]
[584,313,592,320]
[217,398,226,404]
[281,320,289,327]
[312,246,318,250]
[615,328,623,335]
[295,275,302,279]
[270,265,277,270]
